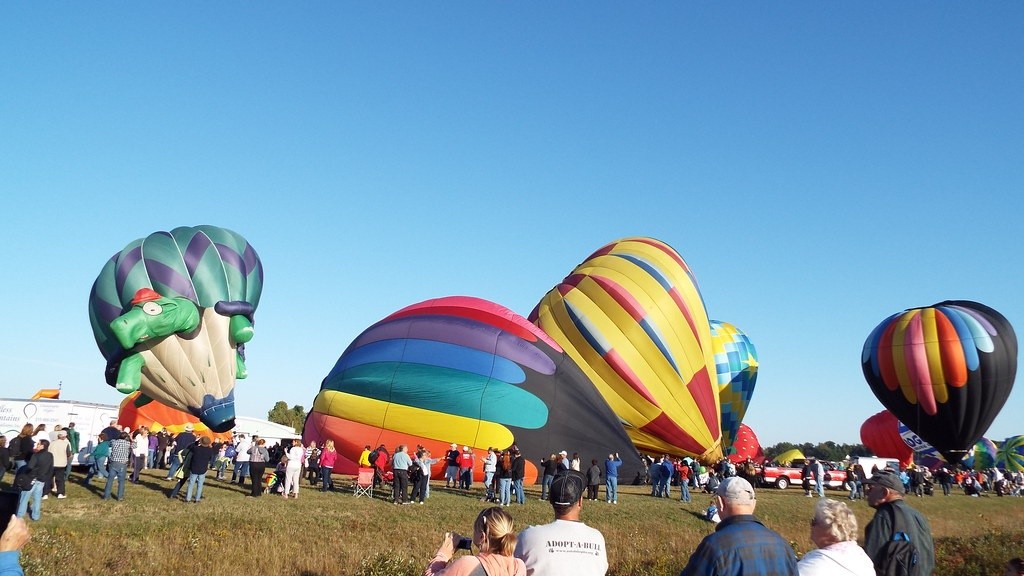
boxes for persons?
[761,458,800,468]
[0,421,80,576]
[796,499,876,576]
[604,452,622,504]
[703,502,722,523]
[801,457,826,498]
[846,460,935,502]
[422,507,528,576]
[862,468,937,576]
[442,443,475,490]
[84,418,338,502]
[679,475,800,575]
[822,460,845,472]
[569,453,581,472]
[480,445,526,508]
[587,459,602,502]
[557,450,569,473]
[639,452,764,503]
[393,443,443,504]
[939,466,1024,499]
[540,453,557,501]
[360,444,389,490]
[517,469,609,576]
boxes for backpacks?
[874,503,918,576]
[8,433,26,457]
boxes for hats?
[559,451,567,455]
[550,470,588,508]
[710,476,756,501]
[862,470,905,493]
[185,420,195,431]
[199,436,212,447]
[463,446,468,452]
[451,444,457,446]
[32,431,49,442]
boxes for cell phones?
[0,490,21,538]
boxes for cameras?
[457,538,472,550]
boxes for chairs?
[352,468,374,499]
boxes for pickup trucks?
[762,458,852,491]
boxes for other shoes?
[58,494,66,499]
[41,496,49,500]
[410,500,415,503]
[420,501,423,504]
[613,501,617,504]
[608,500,611,503]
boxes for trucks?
[851,455,900,480]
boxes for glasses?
[811,517,824,526]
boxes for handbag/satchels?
[176,466,187,479]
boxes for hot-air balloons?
[87,223,265,434]
[994,435,1024,473]
[300,296,649,487]
[860,299,1018,470]
[973,436,999,475]
[525,236,737,478]
[859,409,914,472]
[709,320,759,466]
[898,418,976,478]
[722,423,767,479]
[117,391,232,444]
